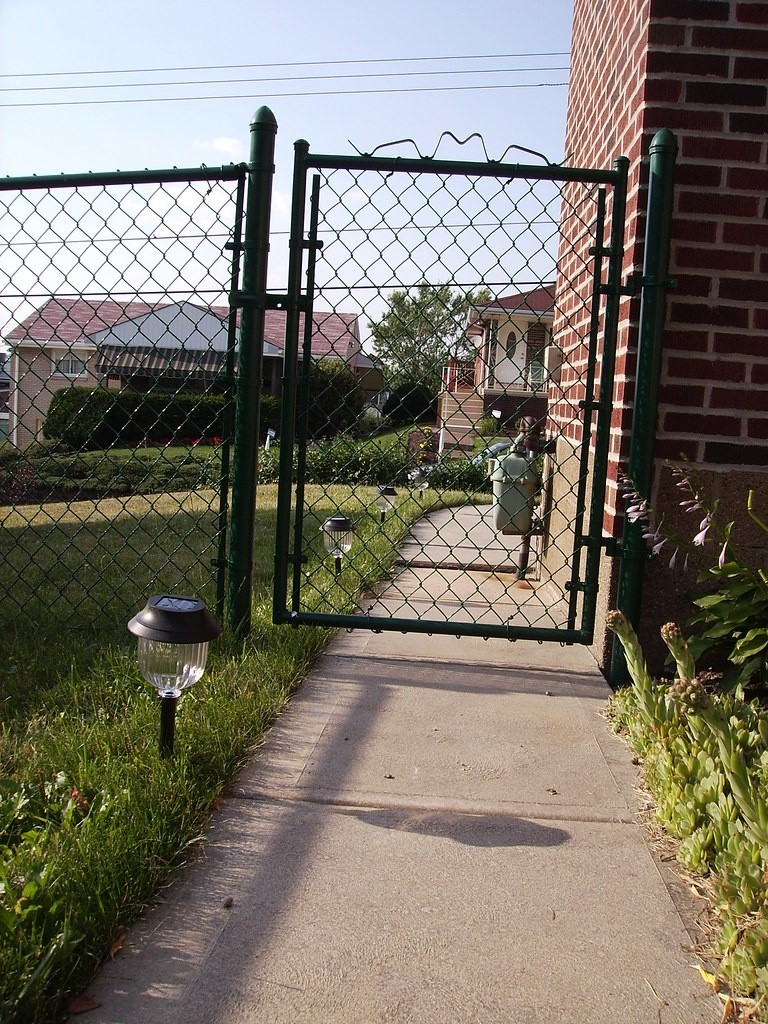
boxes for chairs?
[526,360,546,392]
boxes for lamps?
[127,596,219,700]
[373,485,399,513]
[412,468,431,492]
[318,516,358,560]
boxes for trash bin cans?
[492,456,536,536]
